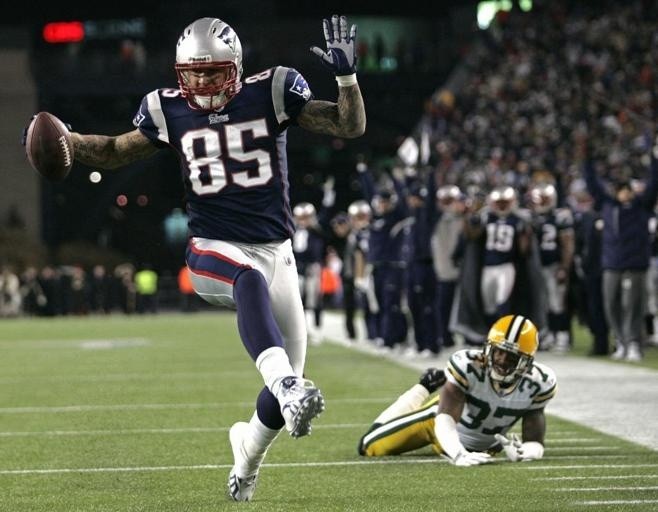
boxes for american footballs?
[26,112,74,185]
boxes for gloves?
[454,449,494,467]
[310,15,358,87]
[494,433,524,463]
[21,115,73,156]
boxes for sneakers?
[550,331,572,353]
[277,376,325,440]
[539,331,554,350]
[227,420,258,502]
[610,339,625,361]
[626,341,642,362]
[418,367,446,393]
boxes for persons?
[2,1,658,360]
[21,16,366,504]
[358,315,558,467]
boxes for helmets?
[348,200,372,231]
[175,17,243,111]
[482,314,539,384]
[293,203,318,229]
[435,185,461,216]
[532,183,558,214]
[489,186,518,218]
